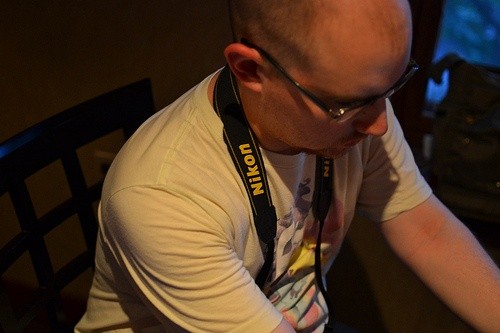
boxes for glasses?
[240,36,418,125]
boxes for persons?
[65,0,500,333]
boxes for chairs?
[0,68,159,333]
